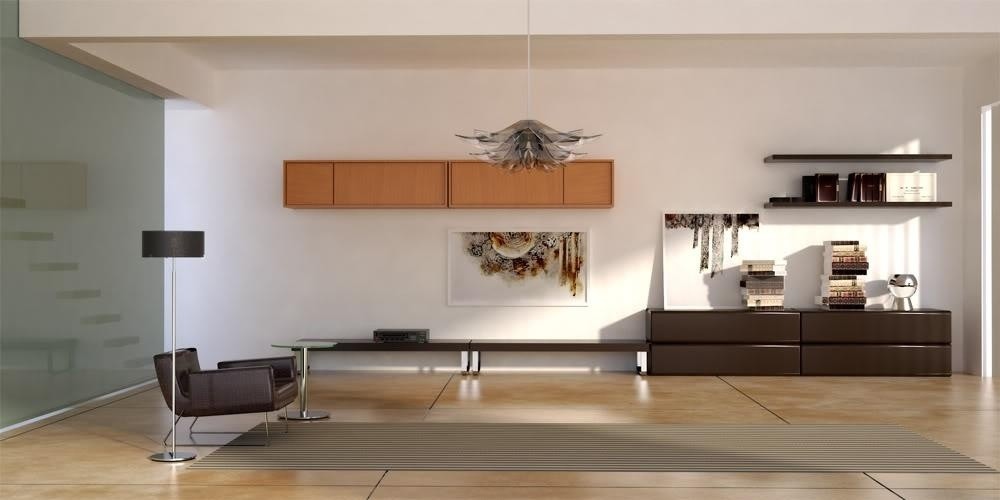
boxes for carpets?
[188,422,1000,473]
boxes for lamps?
[454,0,604,172]
[142,231,204,462]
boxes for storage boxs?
[885,173,937,202]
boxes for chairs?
[154,348,298,446]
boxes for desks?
[271,340,336,420]
[470,339,650,376]
[291,339,472,376]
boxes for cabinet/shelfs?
[763,154,952,207]
[448,160,614,208]
[646,312,801,374]
[801,311,951,374]
[282,160,448,209]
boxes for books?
[801,172,888,202]
[768,197,806,203]
[739,258,789,313]
[814,239,869,311]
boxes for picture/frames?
[447,230,591,307]
[662,209,761,312]
